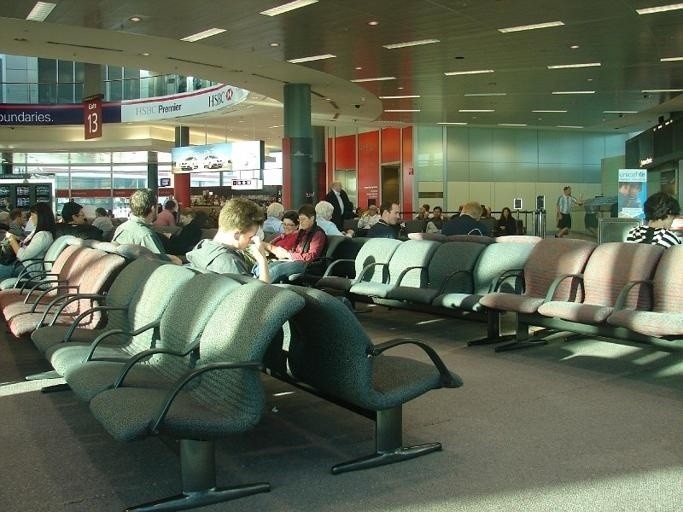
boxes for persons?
[110,189,166,255]
[618,182,643,215]
[324,182,350,231]
[313,201,517,239]
[626,191,681,249]
[1,200,327,277]
[554,186,583,238]
